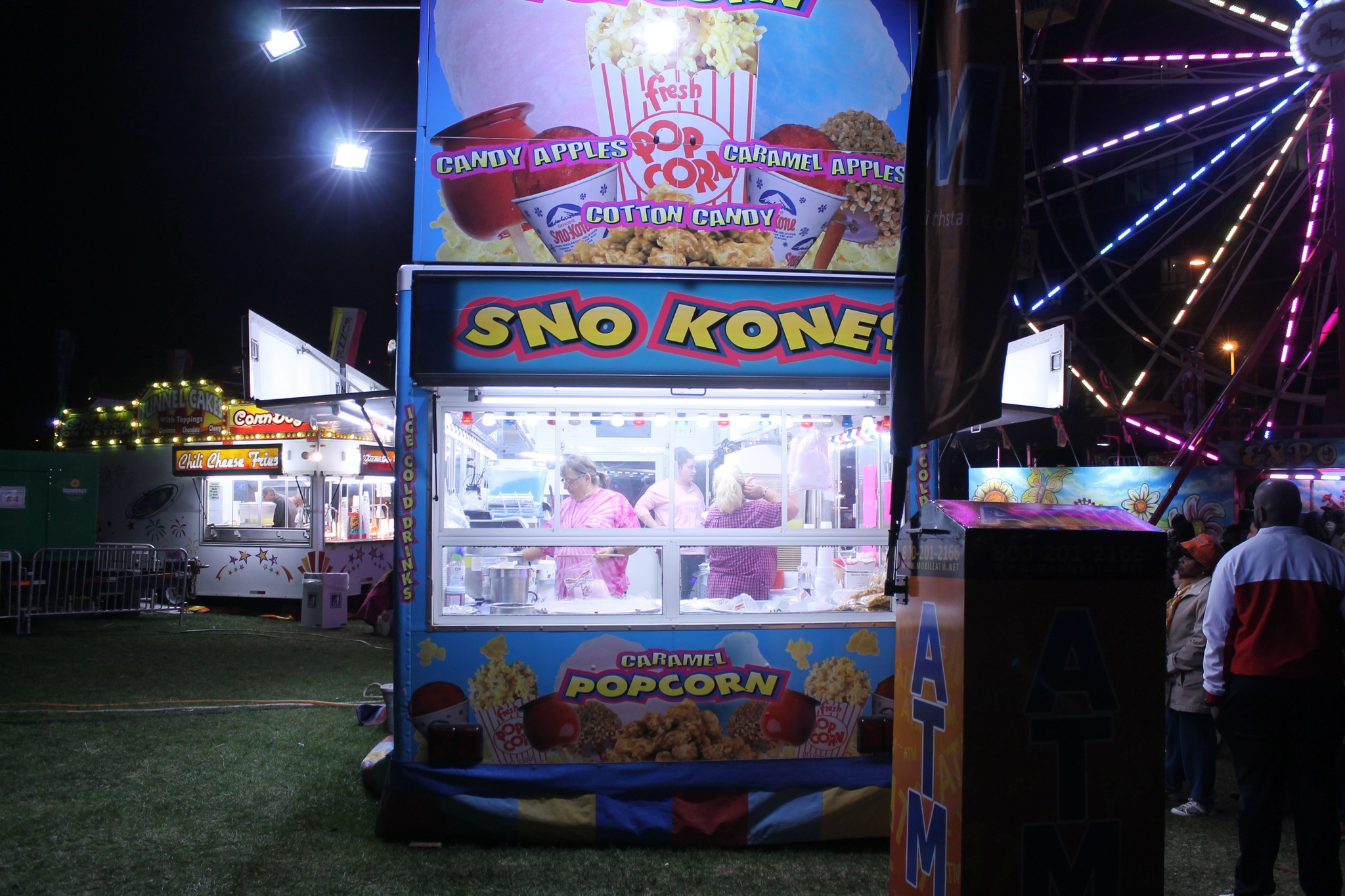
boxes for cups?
[410,698,470,738]
[363,491,370,539]
[801,521,835,597]
[340,497,347,540]
[871,693,894,715]
[746,165,850,268]
[442,546,465,607]
[510,162,620,263]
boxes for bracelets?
[762,487,770,499]
[610,545,618,559]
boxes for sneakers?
[1171,799,1205,815]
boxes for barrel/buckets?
[488,566,531,604]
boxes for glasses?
[561,474,586,490]
[262,493,268,499]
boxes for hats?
[1180,534,1222,570]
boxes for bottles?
[798,562,811,596]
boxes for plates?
[776,546,801,571]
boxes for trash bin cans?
[301,571,351,630]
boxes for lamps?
[257,6,421,64]
[294,507,310,523]
[325,507,340,540]
[330,129,416,175]
[376,504,390,537]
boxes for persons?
[1167,502,1345,842]
[518,454,642,601]
[1201,479,1345,896]
[355,568,393,638]
[262,485,307,528]
[706,463,799,600]
[633,446,709,602]
